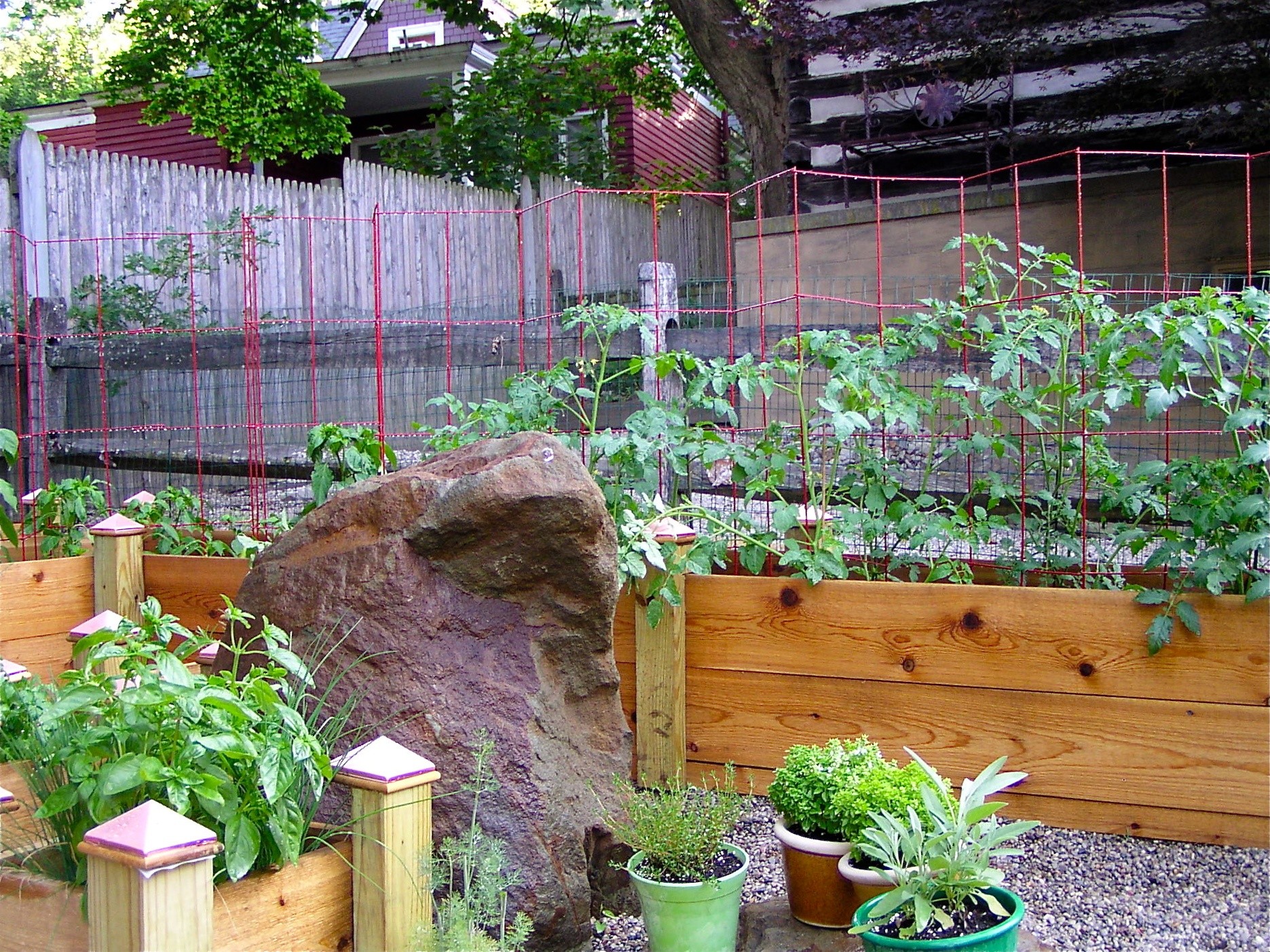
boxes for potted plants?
[3,234,1270,852]
[587,735,1041,952]
